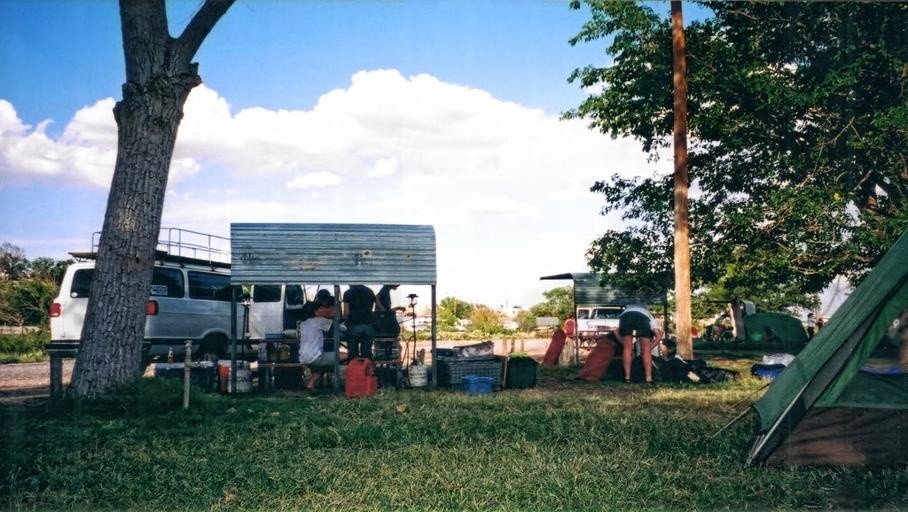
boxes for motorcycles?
[703,324,733,341]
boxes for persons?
[818,317,824,329]
[806,312,815,338]
[711,314,726,342]
[298,282,407,394]
[619,305,661,384]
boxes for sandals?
[623,379,632,383]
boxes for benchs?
[258,361,404,392]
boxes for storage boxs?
[462,375,493,394]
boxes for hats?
[302,300,321,317]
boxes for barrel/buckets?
[217,359,250,394]
[344,356,378,396]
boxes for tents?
[745,227,908,472]
[738,313,810,346]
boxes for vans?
[570,306,626,341]
[45,227,310,373]
